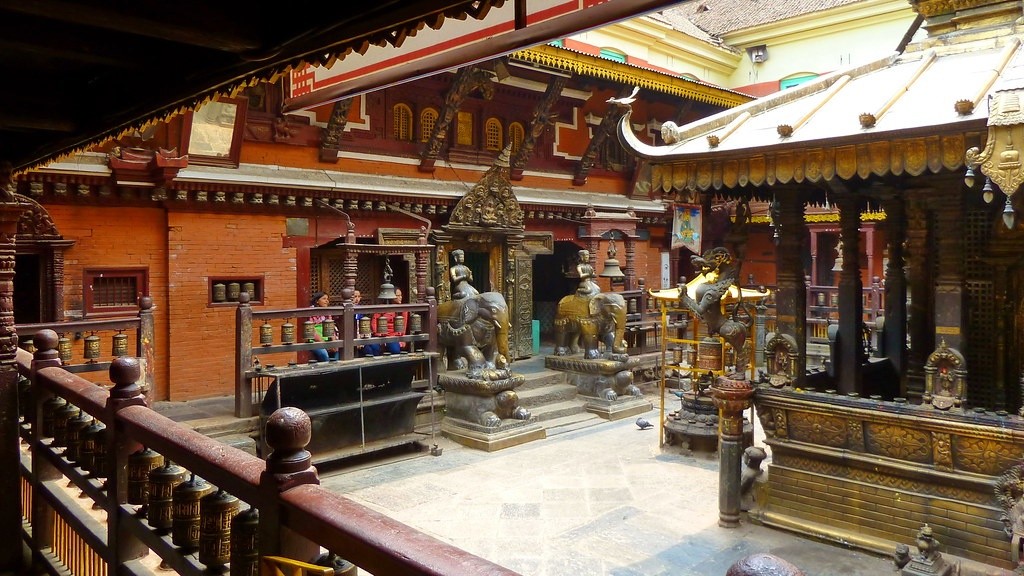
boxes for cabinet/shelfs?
[256,350,441,465]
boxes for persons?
[308,292,338,362]
[372,286,408,353]
[449,249,478,299]
[353,291,378,356]
[576,250,600,296]
[938,368,951,392]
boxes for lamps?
[768,191,781,246]
[964,88,1024,229]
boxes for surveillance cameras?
[751,48,763,63]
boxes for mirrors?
[180,94,249,169]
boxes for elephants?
[551,293,629,362]
[435,291,512,378]
